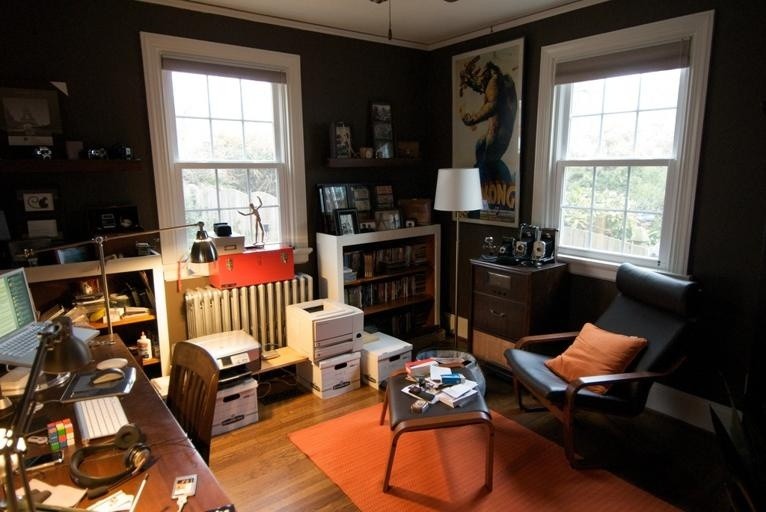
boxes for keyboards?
[74,396,130,443]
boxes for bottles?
[135,332,152,360]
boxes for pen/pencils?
[129,472,150,512]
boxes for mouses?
[90,368,124,385]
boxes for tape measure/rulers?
[410,400,430,414]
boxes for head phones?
[66,425,150,486]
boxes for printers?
[172,330,262,389]
[286,297,364,362]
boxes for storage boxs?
[400,198,436,225]
[362,330,415,393]
[295,350,362,401]
[211,236,245,257]
[205,245,297,291]
[209,377,263,438]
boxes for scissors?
[708,403,766,511]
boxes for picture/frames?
[449,36,527,230]
[14,186,65,241]
[0,87,60,131]
[315,102,426,234]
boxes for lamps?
[432,167,485,352]
[15,220,219,348]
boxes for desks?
[0,332,234,511]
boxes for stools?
[379,362,495,497]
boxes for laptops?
[0,267,99,368]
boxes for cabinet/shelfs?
[0,242,172,397]
[464,256,570,390]
[313,223,444,332]
[0,152,171,401]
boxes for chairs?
[504,262,705,480]
[163,342,220,466]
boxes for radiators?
[182,273,313,351]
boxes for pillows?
[544,319,650,398]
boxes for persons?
[238,196,264,245]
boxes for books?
[344,244,429,309]
[405,357,480,408]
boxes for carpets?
[289,400,682,512]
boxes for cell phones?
[24,451,64,469]
